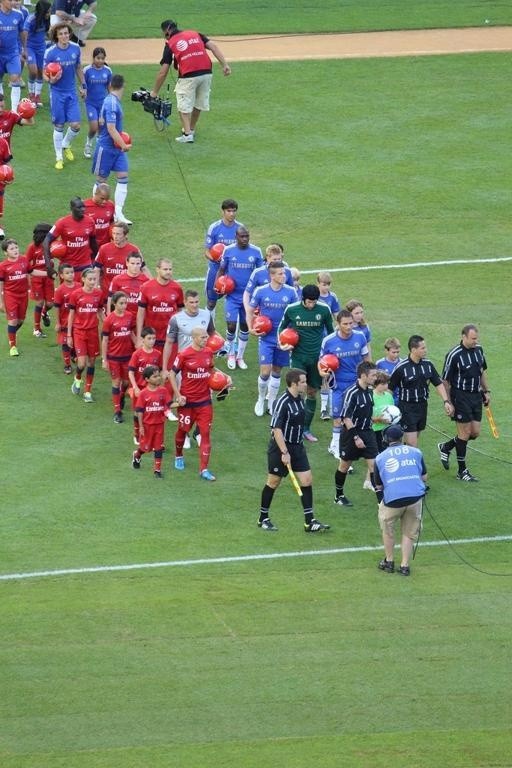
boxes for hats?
[383,426,404,441]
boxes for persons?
[258,368,330,534]
[150,19,231,143]
[1,1,133,238]
[439,324,490,481]
[373,425,428,576]
[1,183,232,481]
[205,200,456,507]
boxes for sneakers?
[33,329,47,338]
[113,412,124,424]
[379,557,395,573]
[398,565,410,575]
[227,355,236,370]
[328,441,338,459]
[237,359,247,369]
[55,160,64,170]
[305,518,330,532]
[64,365,93,402]
[117,216,132,226]
[363,481,374,491]
[10,347,20,356]
[29,93,43,107]
[334,495,353,507]
[42,312,50,327]
[254,401,263,416]
[175,135,194,143]
[257,517,277,531]
[456,469,479,482]
[319,410,331,420]
[132,434,216,481]
[439,442,449,470]
[63,145,74,161]
[181,128,194,136]
[304,431,318,442]
[84,145,92,158]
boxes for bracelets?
[484,391,490,393]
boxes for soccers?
[0,165,13,181]
[320,354,339,370]
[206,335,224,351]
[115,131,130,148]
[211,243,225,261]
[45,62,61,78]
[18,102,35,118]
[253,316,272,334]
[381,406,401,425]
[215,276,235,293]
[50,240,66,257]
[209,372,226,390]
[280,328,298,346]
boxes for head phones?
[381,426,389,447]
